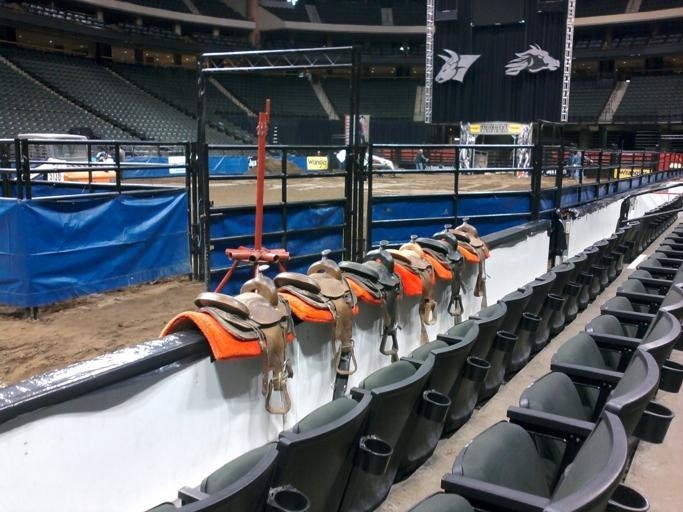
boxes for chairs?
[147,189,683,512]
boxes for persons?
[90,145,126,164]
[415,149,429,170]
[567,151,594,179]
